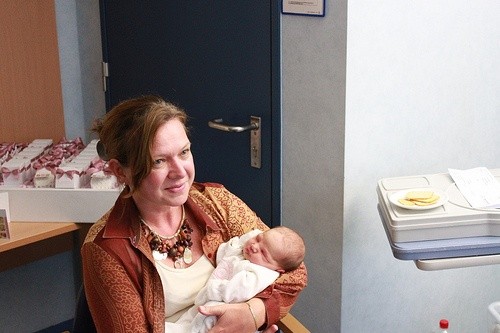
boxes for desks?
[0,223,94,333]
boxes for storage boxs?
[0,188,122,223]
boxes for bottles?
[439,319,449,333]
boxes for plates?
[388,189,450,210]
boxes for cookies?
[398,191,440,205]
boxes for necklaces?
[138,205,193,269]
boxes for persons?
[165,226,306,333]
[80,97,308,333]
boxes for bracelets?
[245,303,258,331]
